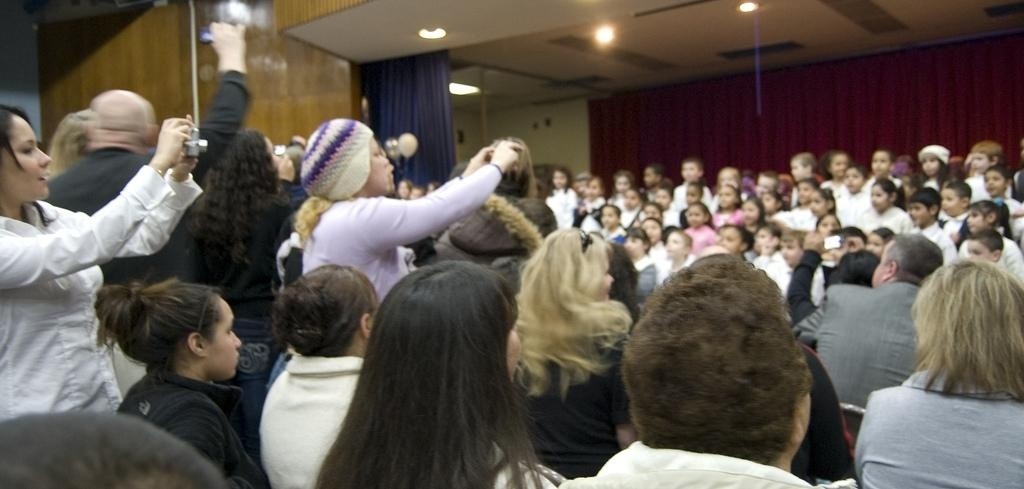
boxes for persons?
[792,232,944,437]
[608,242,640,323]
[397,179,441,267]
[556,254,857,489]
[312,261,567,488]
[189,132,295,475]
[489,137,538,198]
[256,265,379,489]
[45,21,252,398]
[48,109,90,180]
[1,106,203,414]
[853,259,1023,488]
[423,194,557,265]
[94,276,268,488]
[294,117,523,304]
[544,157,824,308]
[787,140,1023,327]
[2,410,235,489]
[508,228,637,480]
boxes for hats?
[918,144,951,166]
[300,118,375,201]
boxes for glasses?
[578,230,592,252]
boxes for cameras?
[183,128,208,157]
[823,234,844,251]
[198,26,214,43]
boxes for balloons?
[384,137,402,162]
[398,134,417,158]
